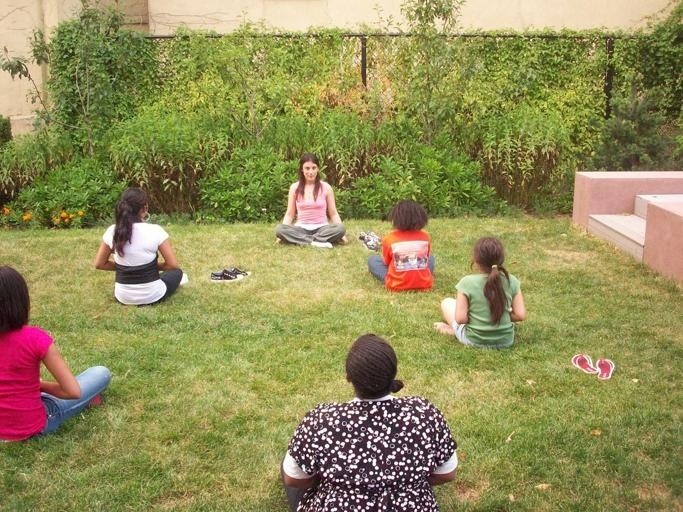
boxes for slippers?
[571,353,614,380]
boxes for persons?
[95,188,188,306]
[275,153,349,248]
[281,334,458,512]
[368,200,435,291]
[0,265,111,442]
[434,237,527,348]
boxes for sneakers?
[358,231,382,252]
[211,268,251,282]
